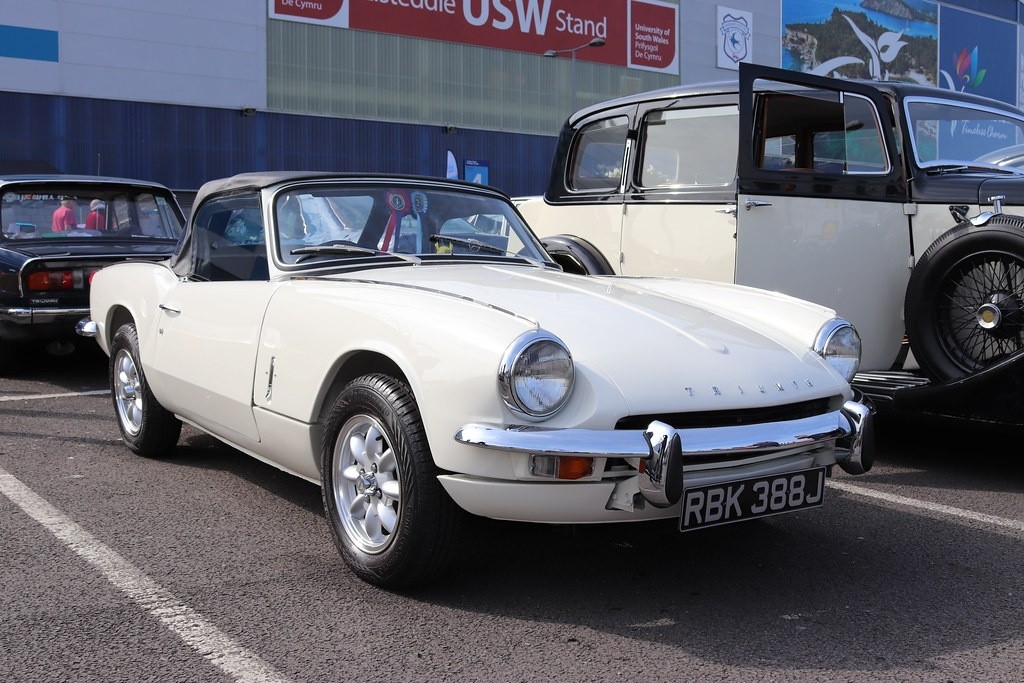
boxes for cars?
[228,193,507,251]
[0,174,189,354]
[503,60,1024,443]
[76,170,879,587]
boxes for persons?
[85,199,114,231]
[52,200,77,231]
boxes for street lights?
[543,38,605,114]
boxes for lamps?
[544,37,606,58]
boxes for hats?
[61,196,77,201]
